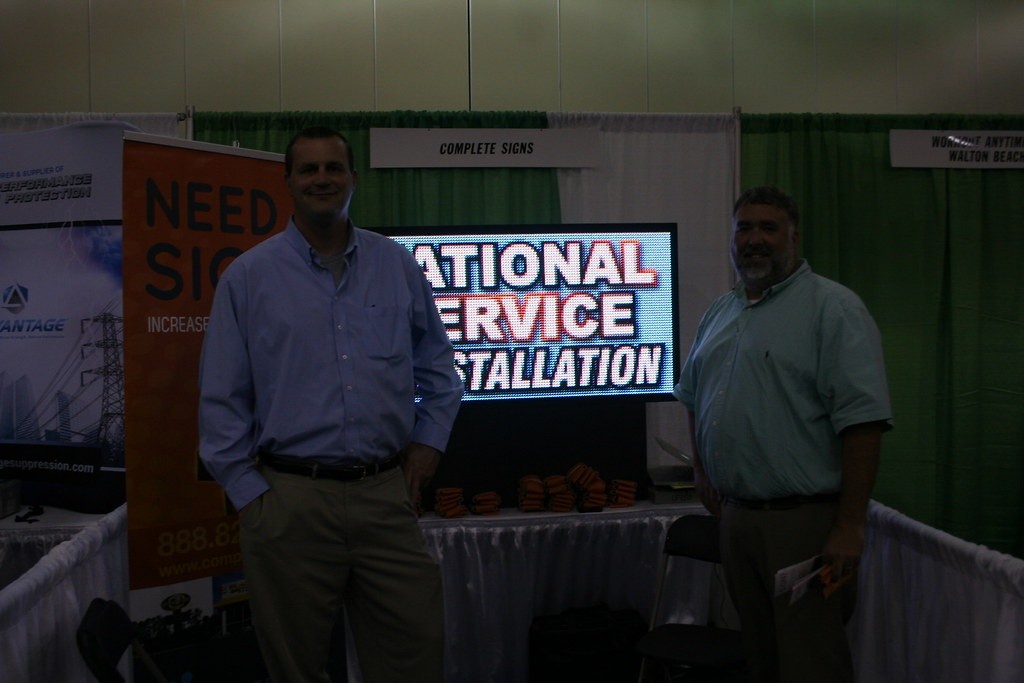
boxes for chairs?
[637,514,764,683]
[75,598,173,683]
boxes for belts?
[259,452,403,481]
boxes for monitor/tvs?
[363,222,681,409]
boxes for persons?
[671,186,894,683]
[194,127,464,683]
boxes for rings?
[844,566,852,573]
